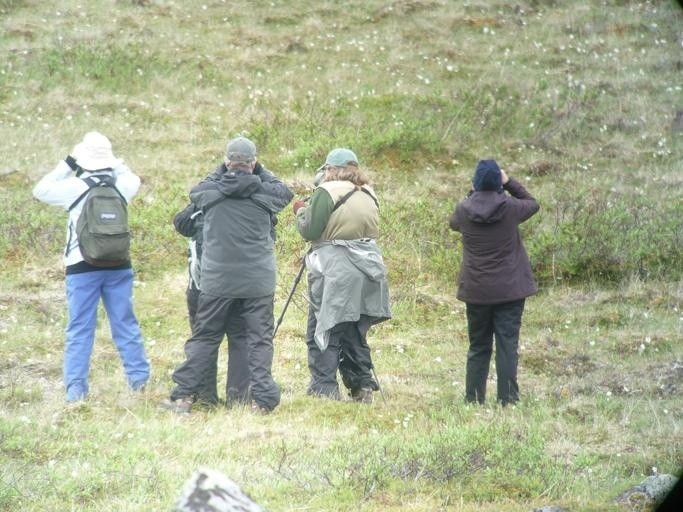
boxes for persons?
[294,148,390,405]
[160,136,293,415]
[449,159,539,406]
[33,131,151,405]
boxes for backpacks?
[72,171,129,267]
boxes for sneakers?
[162,397,191,413]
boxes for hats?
[227,138,255,162]
[76,133,115,170]
[317,149,358,171]
[473,160,501,191]
